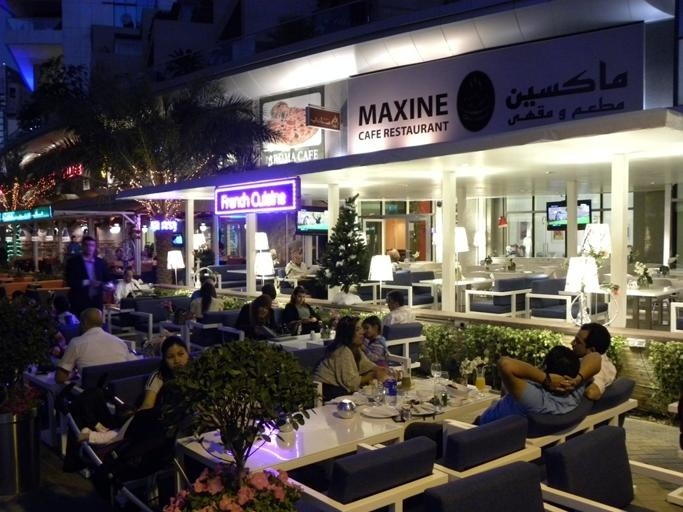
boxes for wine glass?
[431,363,449,406]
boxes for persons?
[571,323,617,401]
[381,290,419,366]
[360,316,391,368]
[137,336,191,412]
[313,316,386,401]
[78,404,135,445]
[404,346,602,460]
[387,248,401,272]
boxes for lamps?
[194,233,208,253]
[255,231,269,252]
[454,226,470,280]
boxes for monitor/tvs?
[171,232,184,247]
[547,200,591,230]
[296,207,328,235]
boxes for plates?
[409,404,436,415]
[361,408,400,419]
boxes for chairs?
[1,250,683,512]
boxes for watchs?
[544,372,552,389]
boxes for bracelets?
[577,373,584,383]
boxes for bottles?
[368,364,415,407]
[474,367,486,392]
[310,324,331,338]
[337,401,357,419]
[279,414,292,432]
